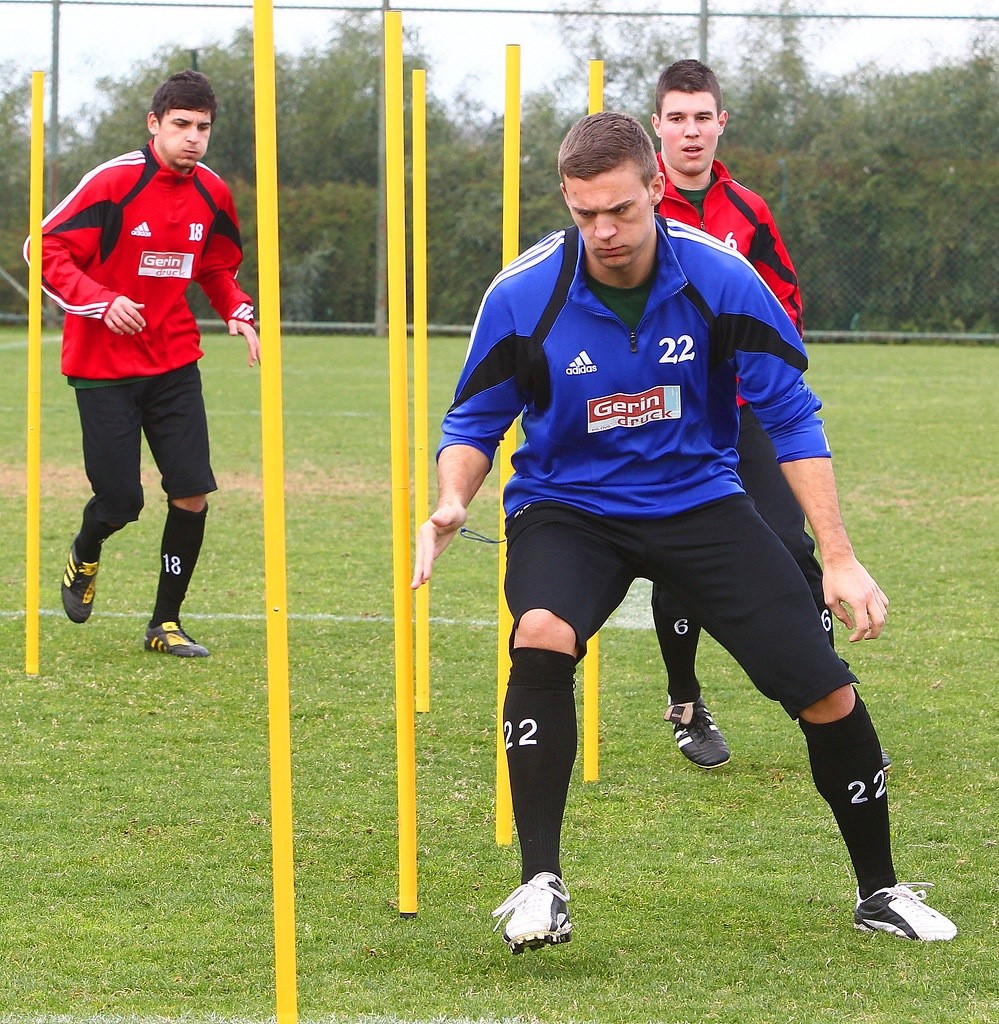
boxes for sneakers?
[143,620,210,658]
[853,882,958,942]
[61,533,101,623]
[881,746,891,771]
[489,872,572,953]
[665,695,733,769]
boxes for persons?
[23,68,261,658]
[653,60,894,774]
[409,114,956,953]
